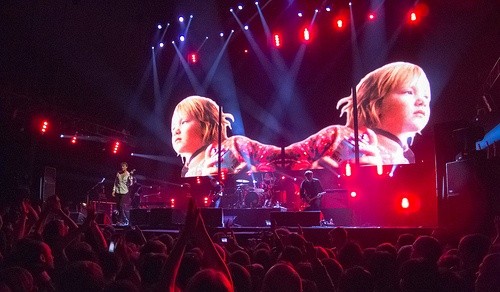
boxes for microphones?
[101,178,105,183]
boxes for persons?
[208,179,223,208]
[112,161,133,227]
[259,172,280,191]
[128,179,152,208]
[300,171,324,210]
[170,96,282,179]
[282,62,431,164]
[178,182,192,210]
[0,192,500,292]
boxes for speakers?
[445,159,476,196]
[152,208,187,228]
[296,211,324,226]
[39,166,57,202]
[128,208,154,227]
[199,207,225,227]
[272,210,297,225]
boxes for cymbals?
[252,171,270,174]
[235,179,250,183]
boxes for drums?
[244,192,264,207]
[236,184,248,191]
[261,193,270,199]
[255,181,266,192]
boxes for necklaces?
[188,144,209,164]
[372,128,402,147]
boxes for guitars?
[311,192,327,201]
[208,188,224,203]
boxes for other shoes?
[122,217,128,222]
